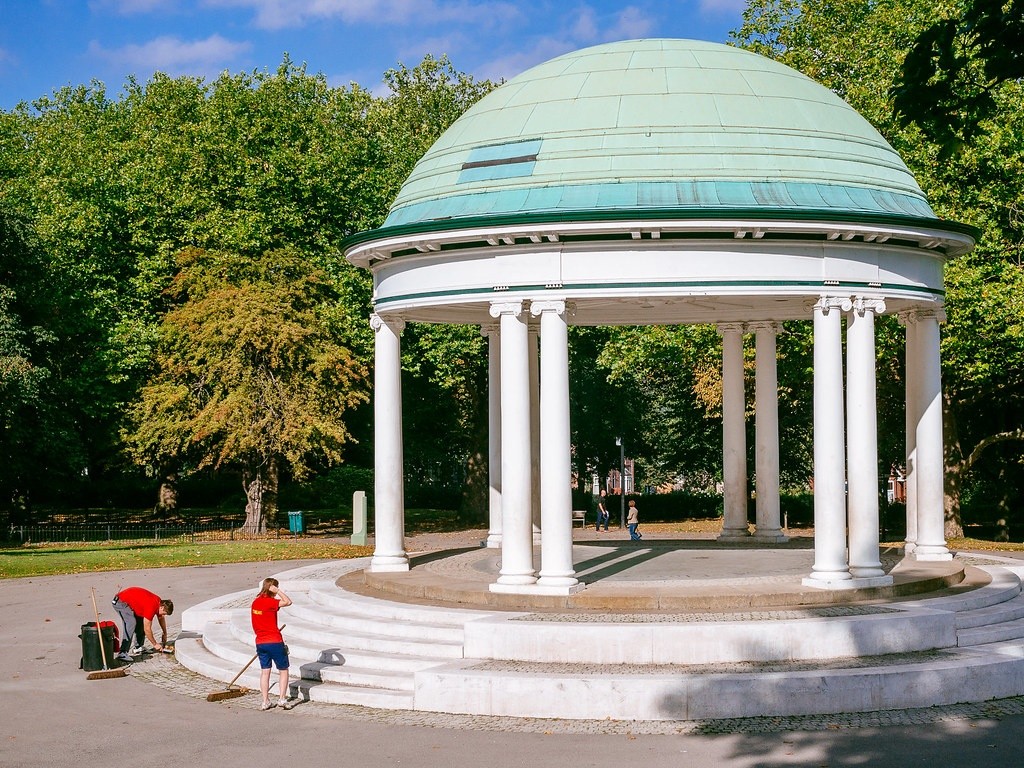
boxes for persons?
[111,587,174,662]
[595,490,610,533]
[250,577,293,711]
[627,500,641,541]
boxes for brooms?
[86,586,127,680]
[206,623,288,701]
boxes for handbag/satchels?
[635,528,642,538]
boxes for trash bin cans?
[289,511,304,533]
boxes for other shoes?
[636,539,641,543]
[115,652,133,661]
[604,530,610,532]
[596,530,601,533]
[134,646,153,654]
[278,699,292,709]
[262,699,278,710]
[629,541,635,544]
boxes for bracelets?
[276,589,279,593]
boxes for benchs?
[572,510,587,529]
